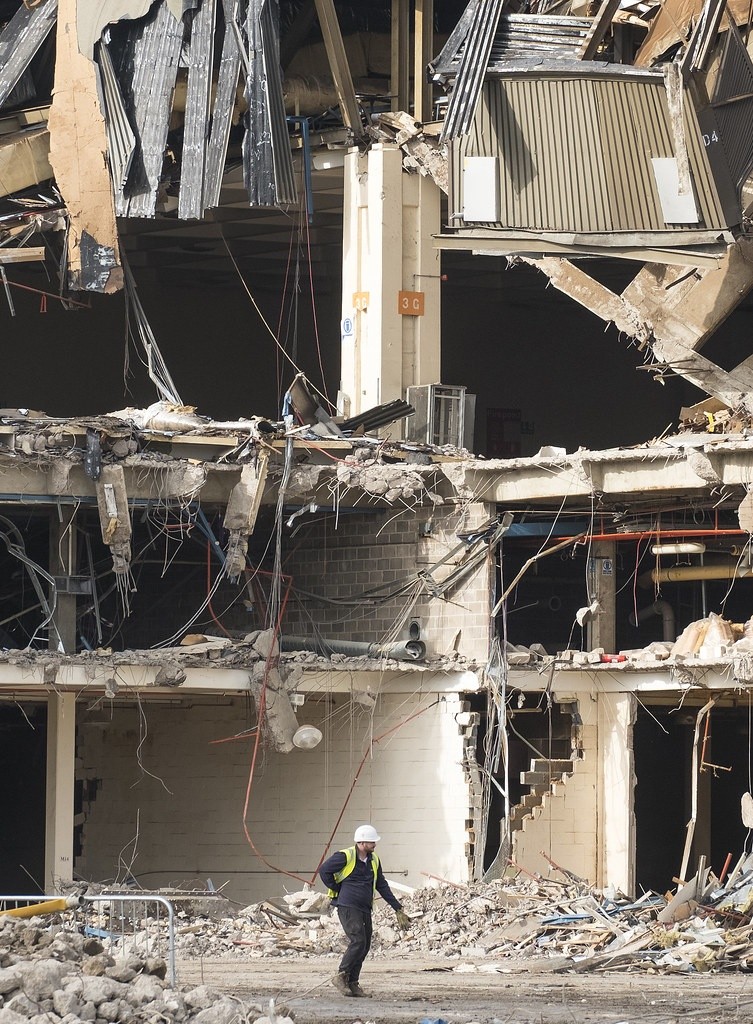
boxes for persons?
[319,825,404,997]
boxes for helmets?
[354,824,381,842]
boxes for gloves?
[395,909,412,932]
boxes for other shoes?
[331,973,353,996]
[346,980,373,998]
